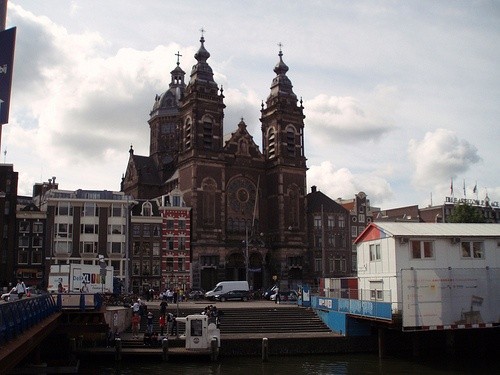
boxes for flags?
[463,178,466,196]
[450,177,453,194]
[473,178,478,193]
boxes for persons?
[202,305,221,329]
[273,286,281,305]
[146,289,154,302]
[13,278,27,300]
[159,287,193,304]
[131,299,178,348]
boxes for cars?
[216,290,252,302]
[1,287,28,300]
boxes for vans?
[204,280,250,301]
